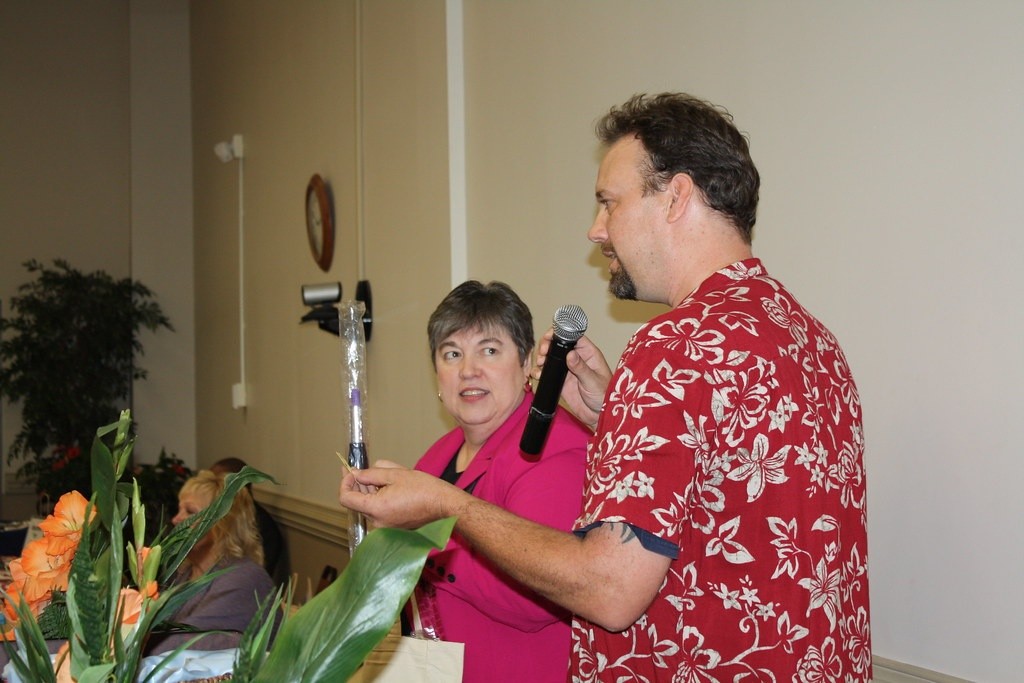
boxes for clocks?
[306,175,334,273]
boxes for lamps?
[301,282,372,341]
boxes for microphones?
[521,304,588,453]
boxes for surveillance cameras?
[302,282,342,306]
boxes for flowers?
[0,408,459,680]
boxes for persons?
[139,469,282,658]
[211,458,290,593]
[339,93,869,683]
[393,280,595,683]
